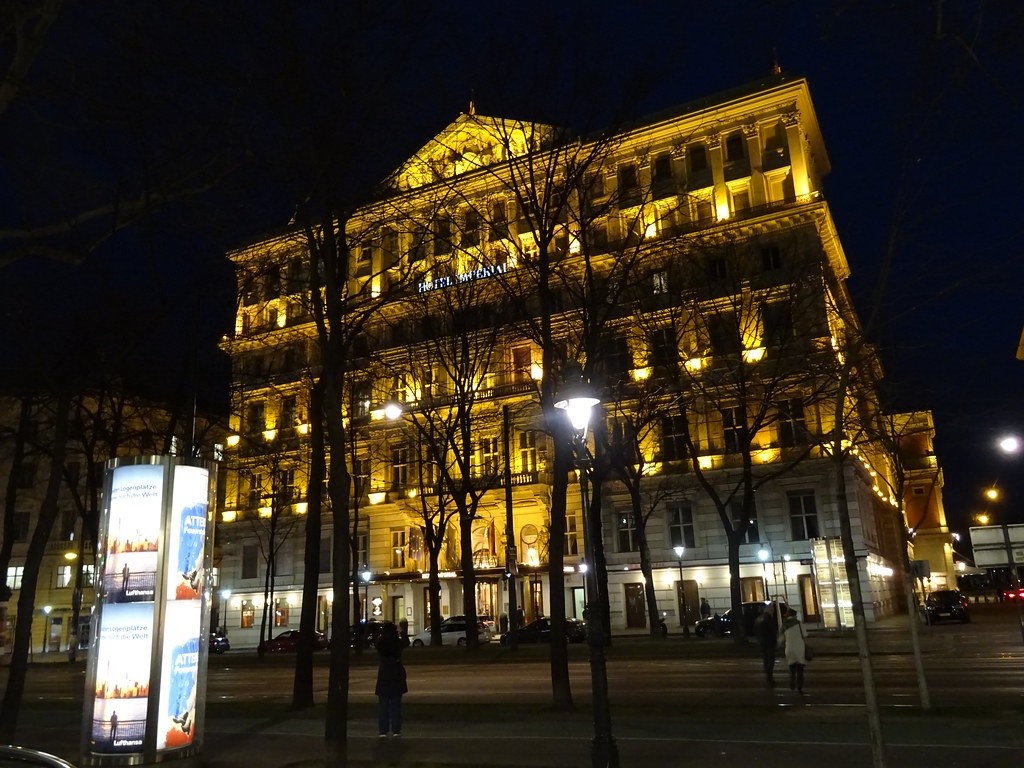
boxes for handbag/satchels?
[805,645,814,661]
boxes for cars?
[925,589,971,625]
[411,614,496,647]
[257,628,330,654]
[351,620,384,646]
[696,600,797,638]
[500,616,588,644]
[209,633,230,654]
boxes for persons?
[700,598,710,618]
[754,604,779,686]
[373,619,411,737]
[122,563,129,591]
[780,608,808,693]
[110,711,117,739]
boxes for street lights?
[758,549,768,600]
[579,557,587,610]
[781,554,790,601]
[361,566,371,621]
[42,605,52,653]
[673,541,690,638]
[552,360,619,768]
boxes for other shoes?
[378,731,386,737]
[394,730,403,736]
